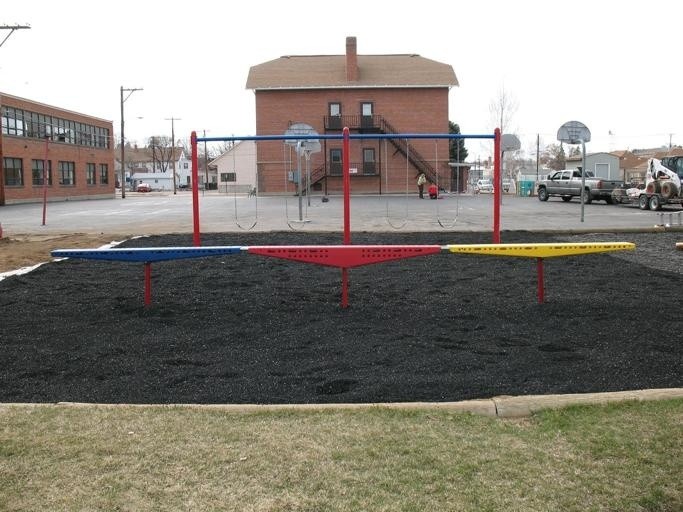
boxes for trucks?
[471,174,510,192]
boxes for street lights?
[667,132,673,148]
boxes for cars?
[136,184,152,192]
[179,184,188,189]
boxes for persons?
[428,182,438,197]
[417,173,426,198]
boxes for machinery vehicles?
[644,155,682,197]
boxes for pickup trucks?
[534,169,627,205]
